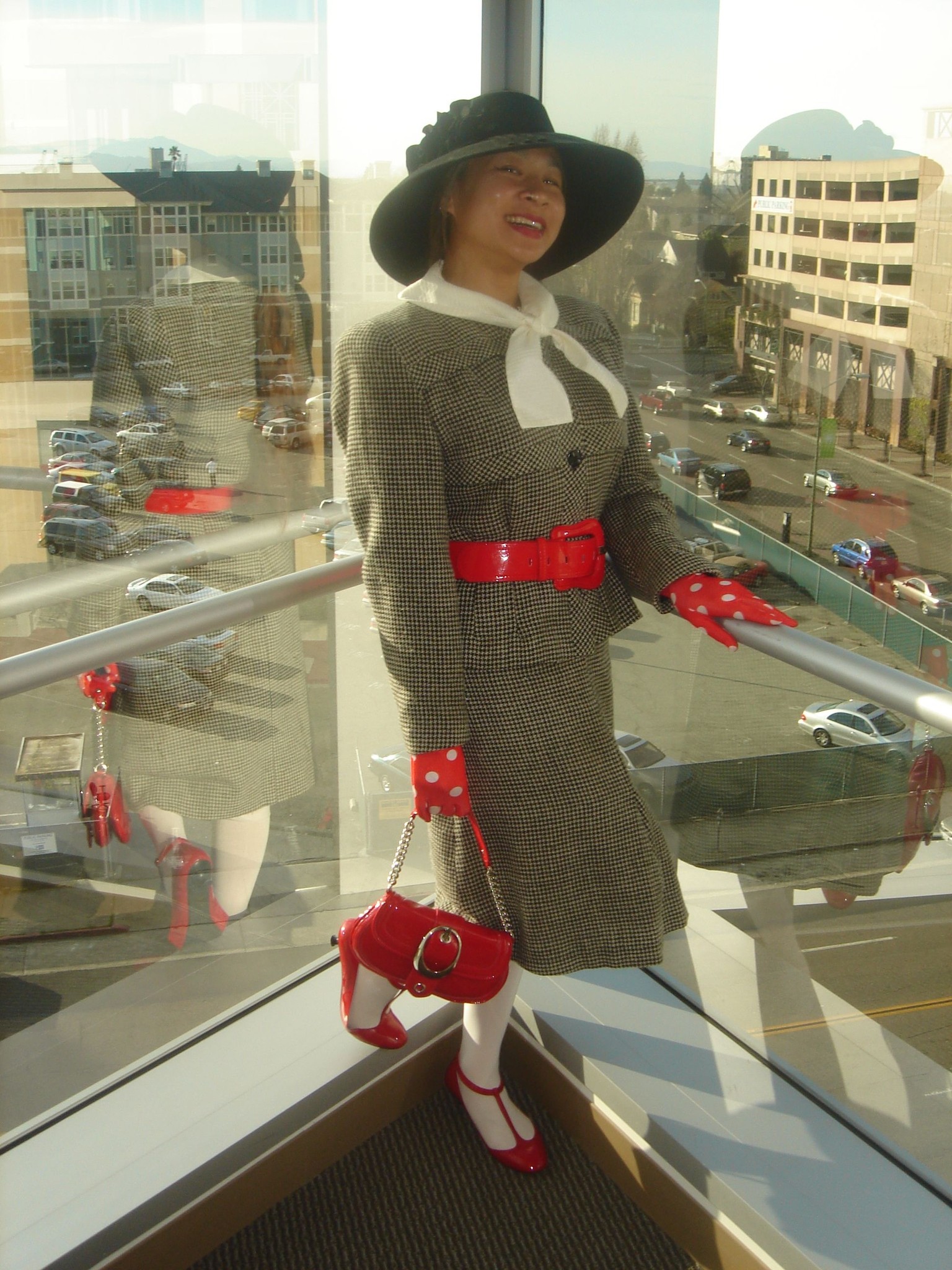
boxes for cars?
[125,573,227,611]
[91,652,215,724]
[133,353,175,370]
[796,698,923,772]
[890,573,952,616]
[367,740,413,791]
[185,629,241,658]
[24,358,71,375]
[158,374,335,451]
[37,401,208,577]
[147,642,229,690]
[680,534,768,589]
[623,361,783,499]
[320,520,364,561]
[803,469,860,498]
[612,728,699,806]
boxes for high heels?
[155,841,215,950]
[448,1054,549,1174]
[330,917,407,1050]
[206,885,246,928]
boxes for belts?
[450,520,611,591]
[138,485,242,518]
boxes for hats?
[108,170,294,223]
[370,91,646,288]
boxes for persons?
[77,140,314,952]
[204,458,218,486]
[333,90,803,1180]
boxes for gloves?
[411,746,472,822]
[78,665,119,711]
[664,571,797,650]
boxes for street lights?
[692,278,705,377]
[806,370,871,559]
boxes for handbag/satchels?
[79,705,129,846]
[353,802,512,1003]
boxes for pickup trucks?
[247,348,292,366]
[300,496,350,534]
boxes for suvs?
[831,535,899,580]
